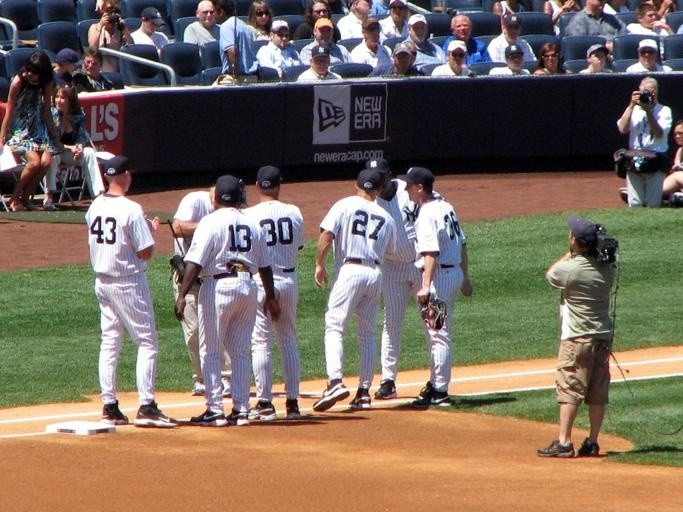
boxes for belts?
[210,271,255,279]
[418,264,455,272]
[345,258,380,267]
[283,265,296,274]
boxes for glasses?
[255,10,270,17]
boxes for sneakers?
[134,406,176,428]
[190,374,232,395]
[375,380,396,398]
[414,388,451,407]
[42,199,56,212]
[312,384,349,411]
[248,403,276,421]
[104,404,128,422]
[350,390,371,410]
[227,410,248,425]
[536,439,575,458]
[190,412,228,426]
[285,400,300,417]
[578,438,600,457]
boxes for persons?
[84,156,179,427]
[537,216,619,458]
[175,176,281,427]
[172,178,246,397]
[239,167,304,422]
[398,167,472,408]
[314,169,395,410]
[366,159,432,398]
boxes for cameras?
[633,156,648,171]
[107,7,125,32]
[639,91,653,102]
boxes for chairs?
[0,1,682,116]
[0,129,96,213]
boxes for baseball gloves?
[421,298,447,330]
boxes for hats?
[271,19,289,33]
[586,40,658,54]
[258,165,279,189]
[55,48,79,65]
[392,40,417,57]
[399,167,436,186]
[105,155,131,175]
[142,6,164,26]
[566,213,598,249]
[311,43,330,58]
[503,16,525,60]
[355,156,390,190]
[362,16,380,31]
[214,175,239,203]
[388,0,427,27]
[446,40,470,56]
[315,17,333,29]
